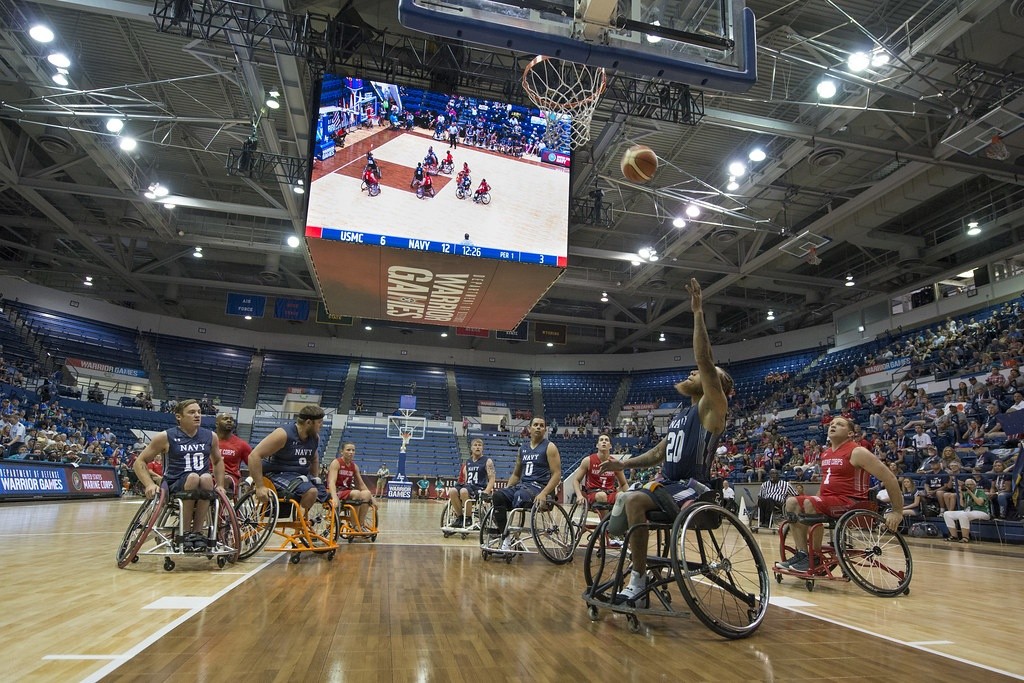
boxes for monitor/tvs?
[23,455,40,461]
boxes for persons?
[450,439,496,528]
[520,426,529,438]
[411,381,415,394]
[550,418,558,438]
[202,394,221,405]
[500,417,507,431]
[416,476,429,499]
[134,399,224,546]
[0,355,177,487]
[776,415,904,573]
[460,233,473,246]
[462,416,468,436]
[424,409,440,420]
[562,302,1024,542]
[374,462,389,498]
[356,399,363,411]
[436,475,445,501]
[492,415,561,550]
[319,463,328,488]
[572,433,629,547]
[333,95,559,204]
[328,442,376,531]
[209,412,253,525]
[248,406,331,522]
[598,277,734,609]
[515,408,532,420]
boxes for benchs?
[0,304,1024,544]
[322,74,569,154]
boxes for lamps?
[659,331,666,342]
[589,189,605,198]
[601,292,608,301]
[106,118,137,151]
[631,205,701,267]
[237,136,259,169]
[767,309,775,320]
[966,217,980,235]
[28,21,73,86]
[267,84,281,110]
[143,181,175,209]
[727,144,767,191]
[845,272,855,287]
[84,276,93,287]
[816,42,890,98]
[293,177,305,194]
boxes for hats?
[382,463,386,465]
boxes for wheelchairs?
[115,468,241,571]
[563,486,639,554]
[772,503,913,599]
[440,488,503,540]
[874,489,940,540]
[716,496,740,520]
[296,480,380,542]
[360,157,491,205]
[154,468,340,565]
[480,483,576,566]
[582,465,771,639]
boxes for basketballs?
[620,145,658,184]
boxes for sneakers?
[450,518,462,526]
[355,526,370,532]
[775,552,808,569]
[609,538,622,546]
[501,540,515,550]
[615,585,651,608]
[459,519,472,526]
[789,554,821,572]
[171,538,192,550]
[192,532,207,550]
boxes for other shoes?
[960,536,971,543]
[944,536,958,541]
